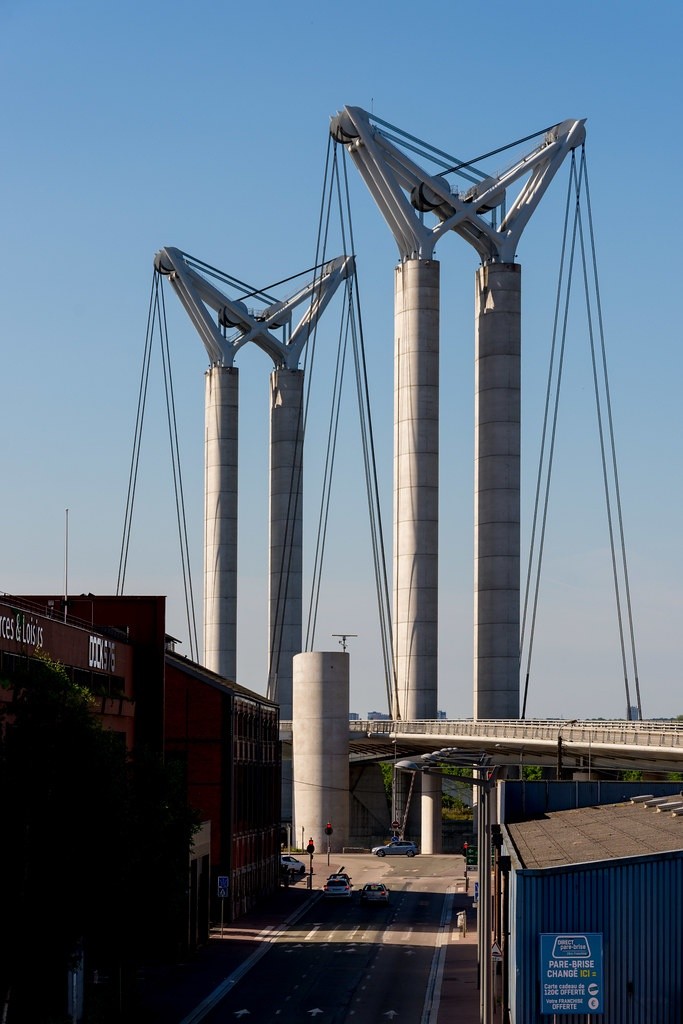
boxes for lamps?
[47,599,55,606]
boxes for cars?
[280,854,306,875]
[326,873,352,885]
[323,878,354,902]
[359,883,391,906]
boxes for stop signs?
[392,821,400,828]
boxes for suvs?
[372,840,421,858]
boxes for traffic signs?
[466,846,495,856]
[466,854,495,866]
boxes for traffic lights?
[327,823,331,834]
[309,840,313,853]
[463,841,469,857]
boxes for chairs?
[377,887,382,891]
[367,886,371,890]
